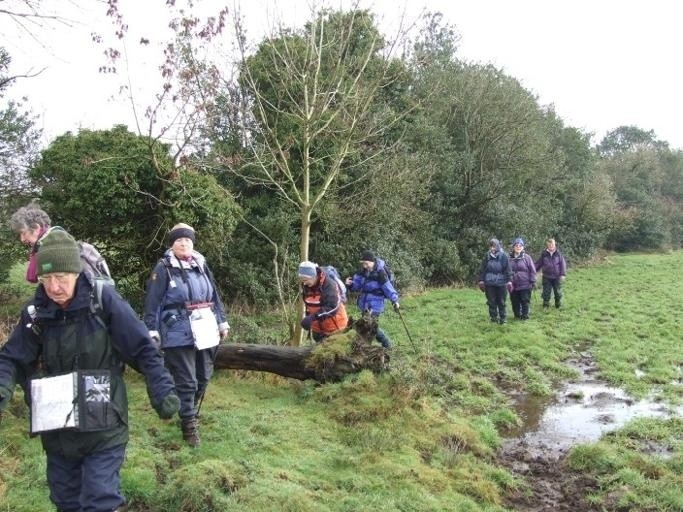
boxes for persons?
[144,222,232,448]
[478,237,514,326]
[344,249,400,351]
[505,236,537,321]
[0,228,184,512]
[534,238,567,313]
[297,260,348,343]
[8,204,75,286]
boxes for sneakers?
[179,424,201,446]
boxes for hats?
[511,238,524,247]
[489,238,500,248]
[298,260,317,279]
[167,222,196,246]
[358,250,374,263]
[34,226,82,275]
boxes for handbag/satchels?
[322,265,347,305]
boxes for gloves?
[531,282,538,291]
[505,281,513,293]
[158,394,182,419]
[300,314,316,331]
[391,300,400,313]
[478,280,485,293]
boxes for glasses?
[37,273,79,284]
